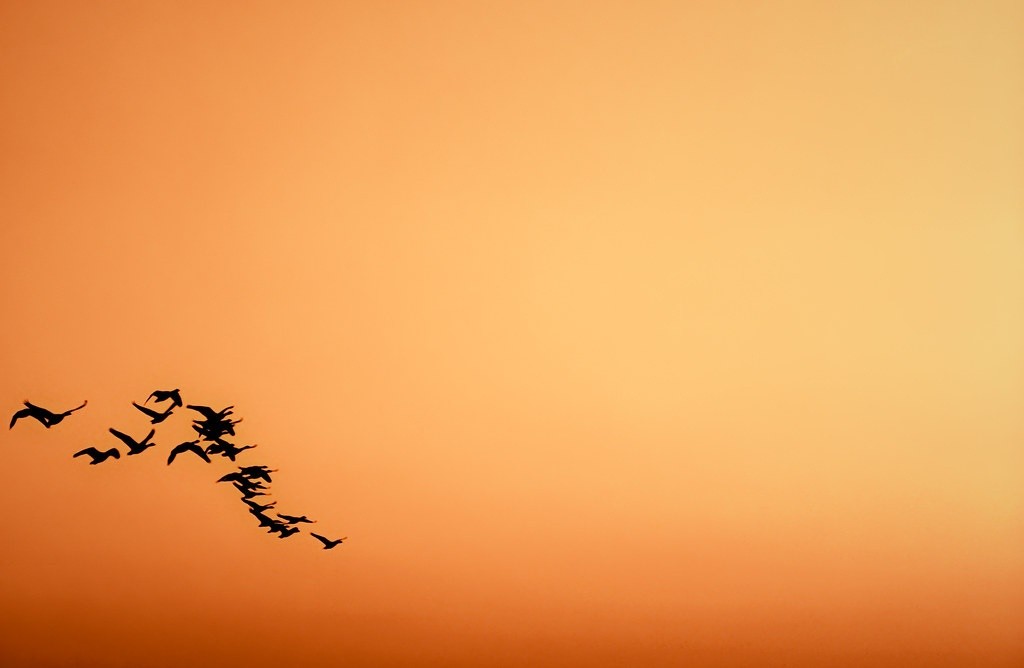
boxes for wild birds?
[7,400,91,430]
[73,389,349,551]
